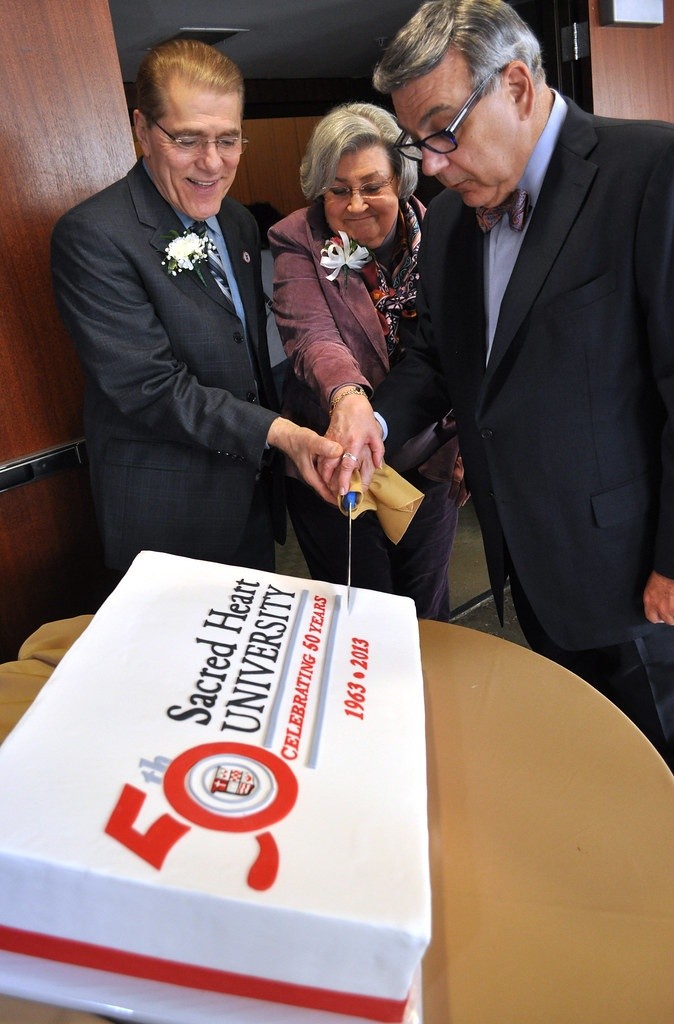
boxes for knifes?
[343,492,356,608]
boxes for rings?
[342,453,358,462]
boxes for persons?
[266,101,472,624]
[317,0,674,775]
[50,37,343,614]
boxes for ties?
[189,221,233,305]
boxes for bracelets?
[328,386,369,418]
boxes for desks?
[0,604,673,1024]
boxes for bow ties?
[475,189,533,234]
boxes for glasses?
[321,169,398,202]
[147,113,248,155]
[393,70,501,162]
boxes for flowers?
[158,227,216,285]
[322,230,373,283]
